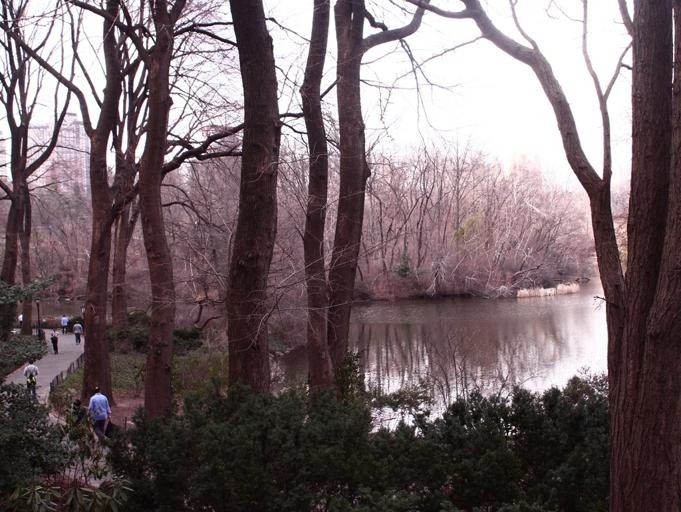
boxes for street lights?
[80,305,86,320]
[36,298,40,338]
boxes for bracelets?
[108,416,111,419]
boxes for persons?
[23,359,38,397]
[16,312,23,328]
[87,387,112,445]
[61,314,68,334]
[73,320,83,345]
[50,328,59,354]
[66,399,92,455]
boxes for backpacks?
[27,365,36,386]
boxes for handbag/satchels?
[104,422,115,438]
